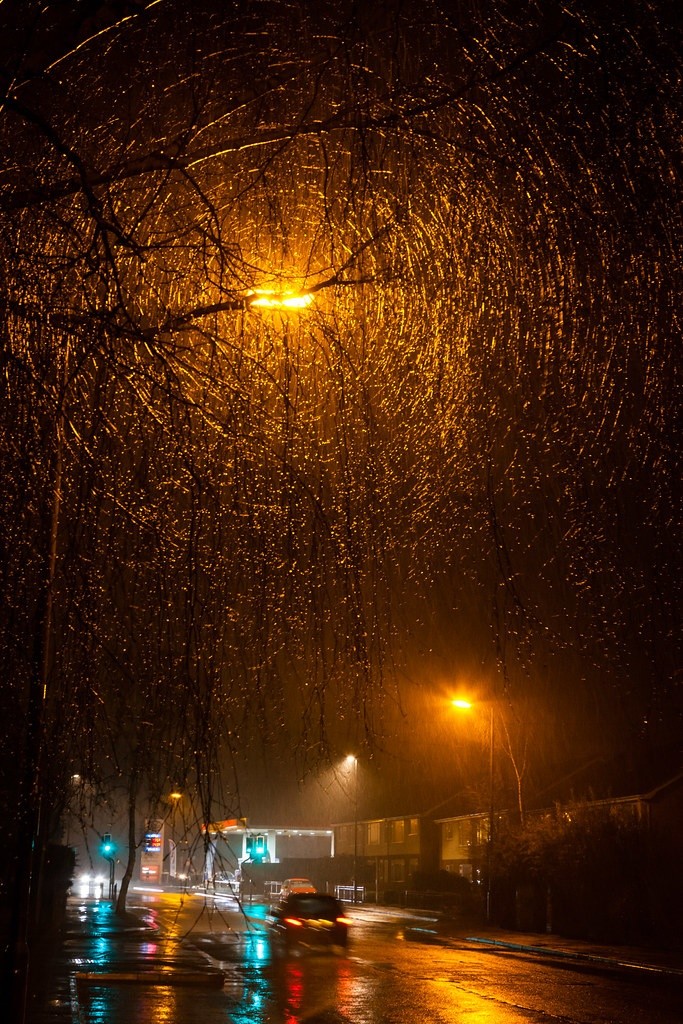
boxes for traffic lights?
[104,834,111,851]
[256,836,264,853]
[246,838,253,853]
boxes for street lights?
[170,793,181,841]
[453,699,495,921]
[4,289,309,1024]
[346,755,357,904]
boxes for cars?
[267,893,347,950]
[279,878,317,902]
[213,871,235,881]
